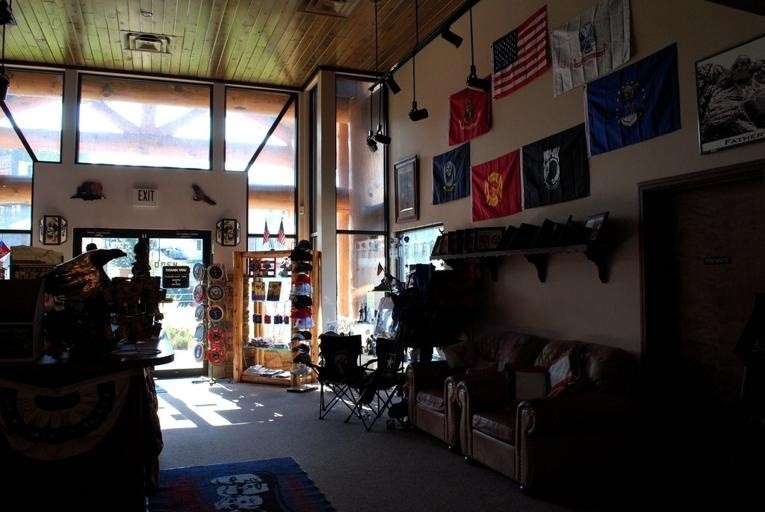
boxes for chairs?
[317,334,364,419]
[344,338,406,432]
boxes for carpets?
[147,456,335,512]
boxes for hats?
[292,240,313,374]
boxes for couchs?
[405,332,548,452]
[455,339,635,492]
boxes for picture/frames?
[455,230,463,254]
[439,233,448,255]
[583,211,610,241]
[448,231,456,255]
[431,236,442,256]
[393,154,419,224]
[473,227,506,252]
[694,34,765,155]
[464,228,474,253]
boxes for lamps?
[384,58,404,94]
[441,23,464,48]
[367,91,378,152]
[409,0,428,122]
[374,81,391,144]
[466,7,492,92]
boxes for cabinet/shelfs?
[233,251,319,387]
[637,158,765,512]
[1,280,46,361]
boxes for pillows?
[506,363,551,405]
[546,346,577,390]
[442,339,478,369]
[548,386,565,397]
[467,362,498,376]
[494,338,522,373]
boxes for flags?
[521,123,592,210]
[432,142,470,206]
[448,74,493,146]
[278,221,285,246]
[548,0,631,97]
[262,221,269,244]
[490,2,551,102]
[583,41,682,157]
[470,148,524,223]
